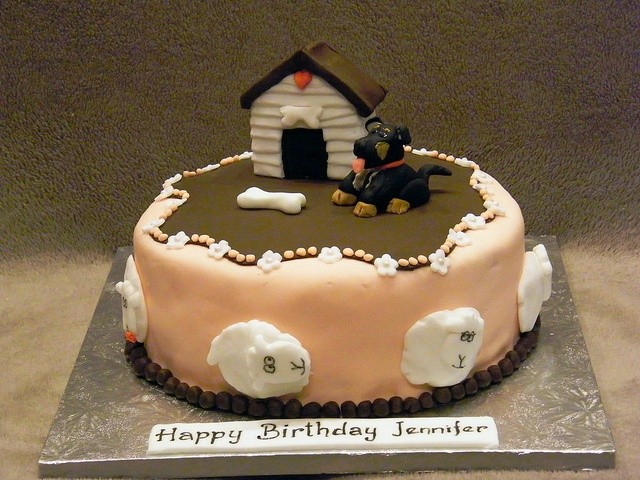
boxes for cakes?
[114,41,552,419]
